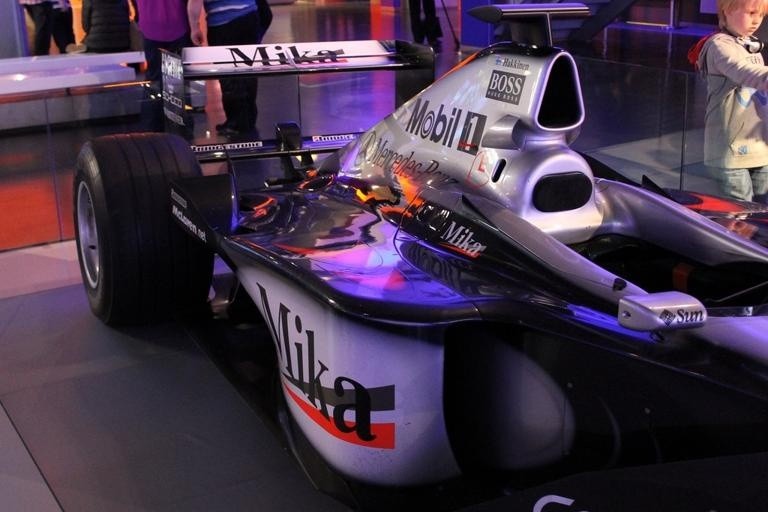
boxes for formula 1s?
[72,3,768,510]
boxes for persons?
[17,1,78,57]
[132,1,196,144]
[698,1,767,205]
[80,1,129,54]
[407,1,444,57]
[185,1,274,143]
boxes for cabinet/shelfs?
[0,49,148,253]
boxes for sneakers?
[215,119,244,132]
